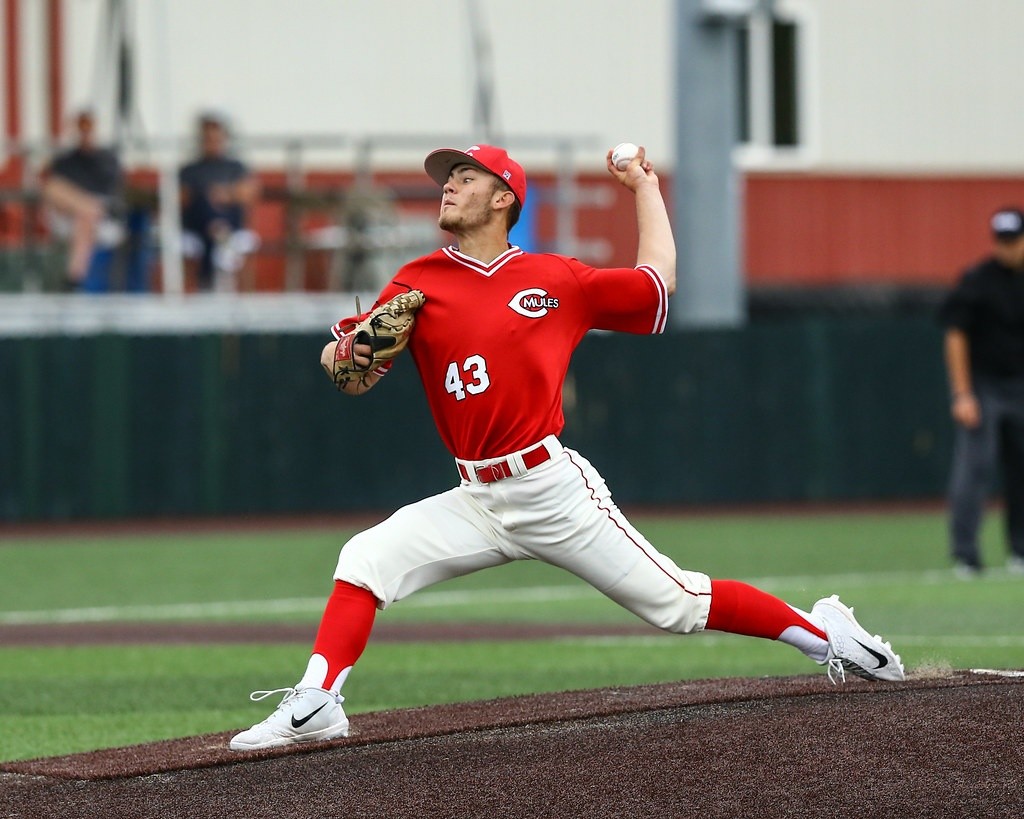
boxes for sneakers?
[1005,557,1024,576]
[955,559,989,582]
[811,594,906,686]
[230,685,349,751]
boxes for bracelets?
[946,387,974,398]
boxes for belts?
[458,445,550,482]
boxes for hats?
[424,144,526,212]
[991,210,1024,238]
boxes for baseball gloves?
[332,289,426,383]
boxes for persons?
[228,143,907,752]
[935,202,1023,582]
[36,107,133,293]
[178,115,261,294]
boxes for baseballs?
[611,142,638,171]
